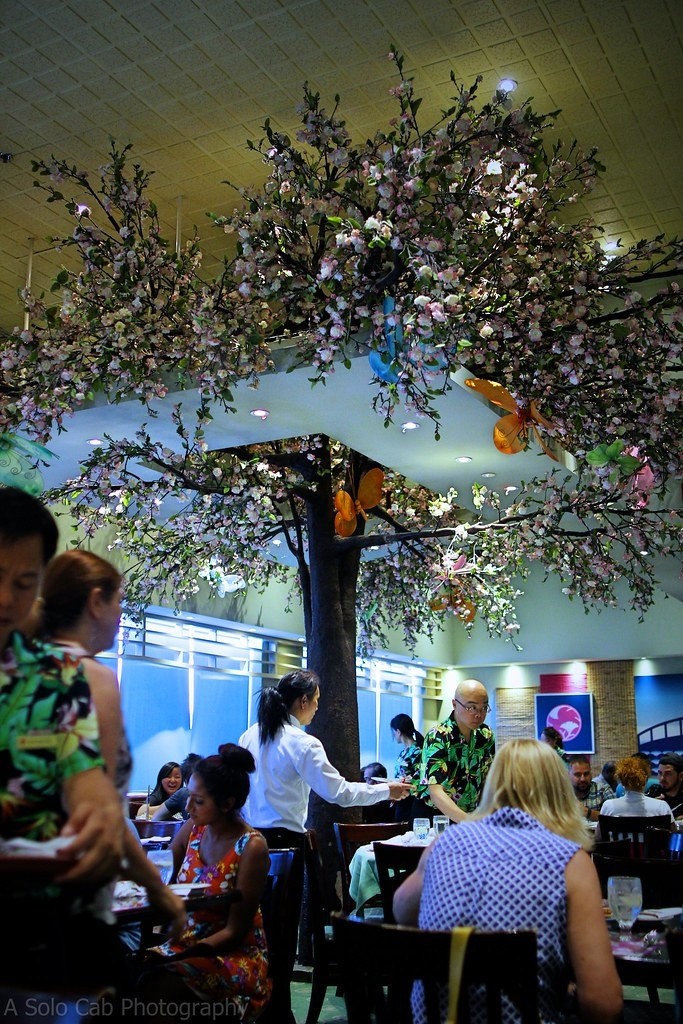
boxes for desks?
[104,882,244,1024]
[603,905,683,1023]
[351,822,464,923]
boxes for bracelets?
[587,808,591,819]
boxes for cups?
[412,818,430,841]
[432,816,449,835]
[147,849,173,887]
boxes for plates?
[371,777,411,782]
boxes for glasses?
[455,699,492,712]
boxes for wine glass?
[607,876,642,942]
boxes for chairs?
[326,916,540,1024]
[245,842,304,1023]
[123,818,683,915]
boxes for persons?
[393,738,622,1024]
[0,486,187,996]
[136,762,184,816]
[615,753,660,798]
[595,759,678,860]
[390,713,424,824]
[81,743,270,1024]
[568,754,615,822]
[412,679,495,825]
[238,670,416,1024]
[363,763,390,824]
[645,753,683,819]
[541,728,571,768]
[151,753,201,822]
[592,761,618,793]
